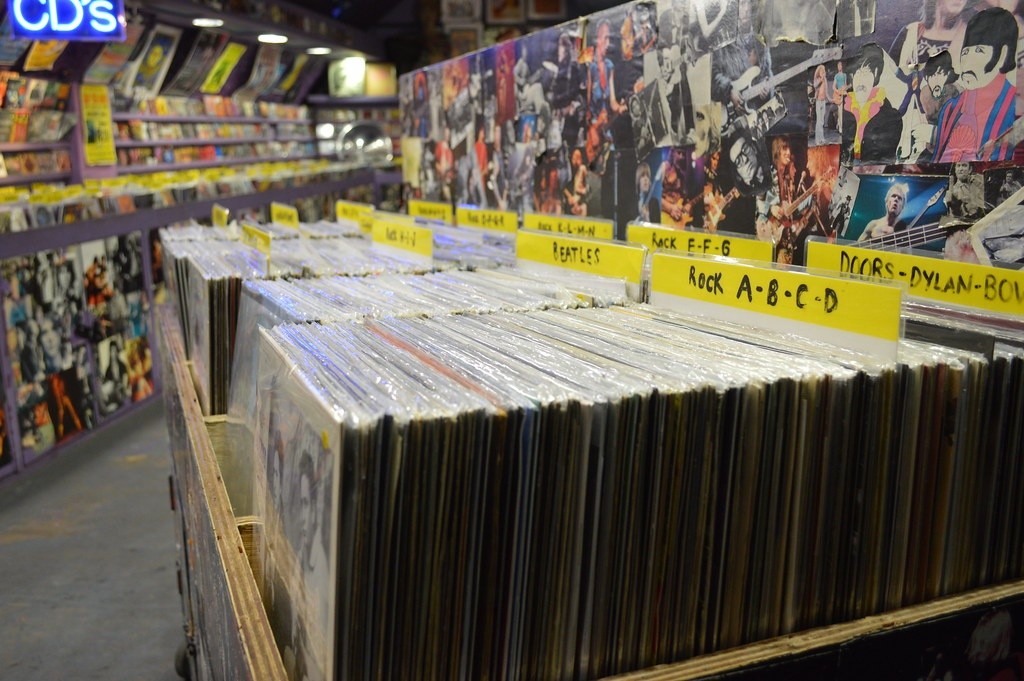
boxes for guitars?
[585,77,644,178]
[705,187,740,232]
[658,45,714,97]
[756,164,837,248]
[660,190,704,230]
[843,221,949,251]
[905,182,947,231]
[715,46,844,138]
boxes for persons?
[0,221,167,457]
[405,0,1024,265]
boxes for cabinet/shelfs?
[0,0,1024,681]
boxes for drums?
[539,61,557,92]
